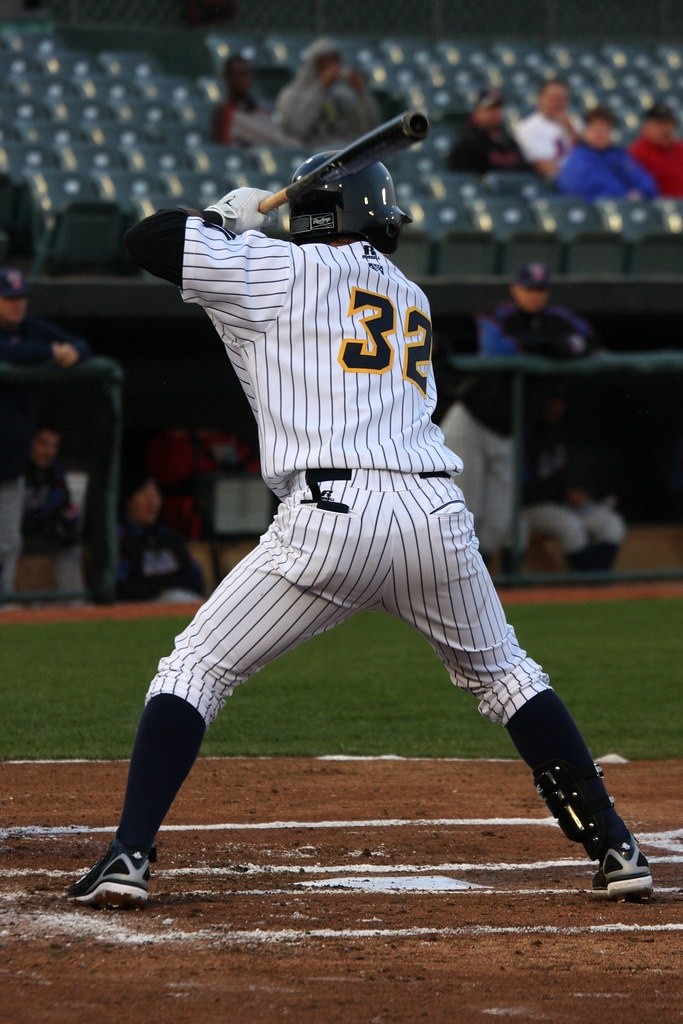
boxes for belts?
[305,468,452,486]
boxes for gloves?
[203,187,278,235]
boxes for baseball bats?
[257,113,431,213]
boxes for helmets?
[289,150,413,255]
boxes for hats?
[519,261,550,290]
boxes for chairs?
[0,26,683,280]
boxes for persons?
[0,266,282,603]
[211,35,381,149]
[58,150,650,899]
[437,260,628,574]
[438,77,683,200]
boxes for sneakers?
[67,837,150,909]
[591,832,654,903]
